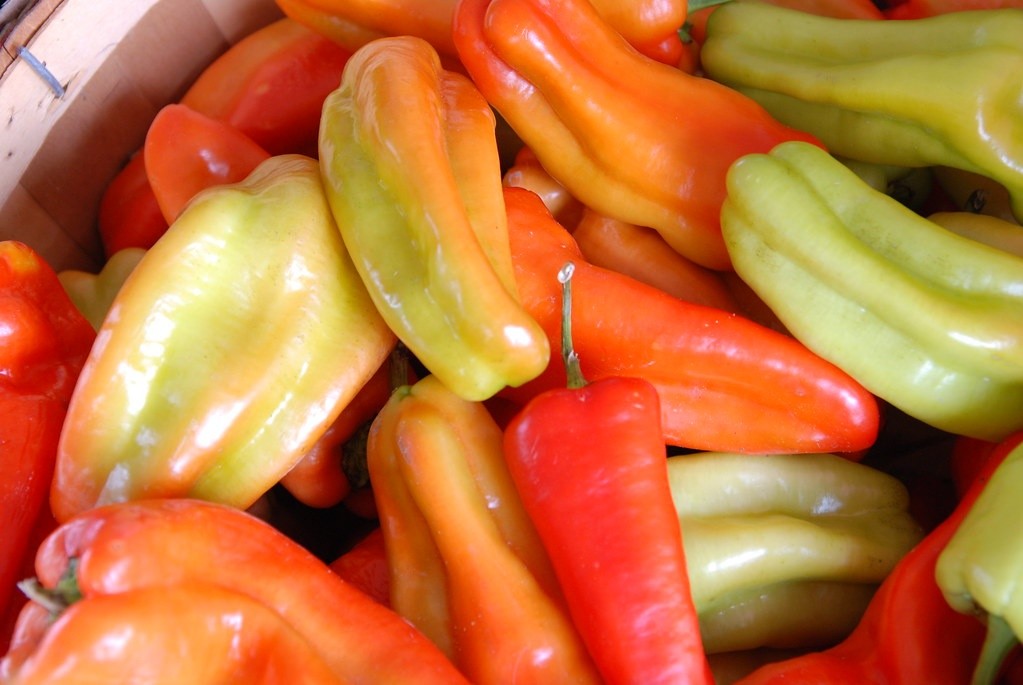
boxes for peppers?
[0,0,1023,685]
